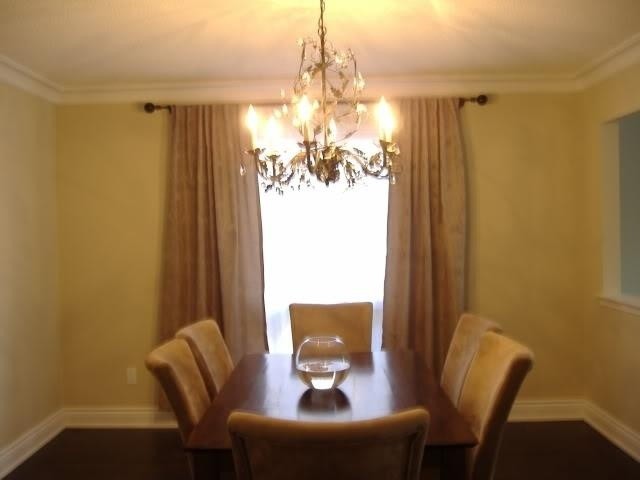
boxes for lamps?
[239,1,405,194]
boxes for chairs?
[144,339,212,480]
[289,302,373,354]
[439,315,504,409]
[458,332,535,480]
[176,320,235,402]
[226,409,430,480]
